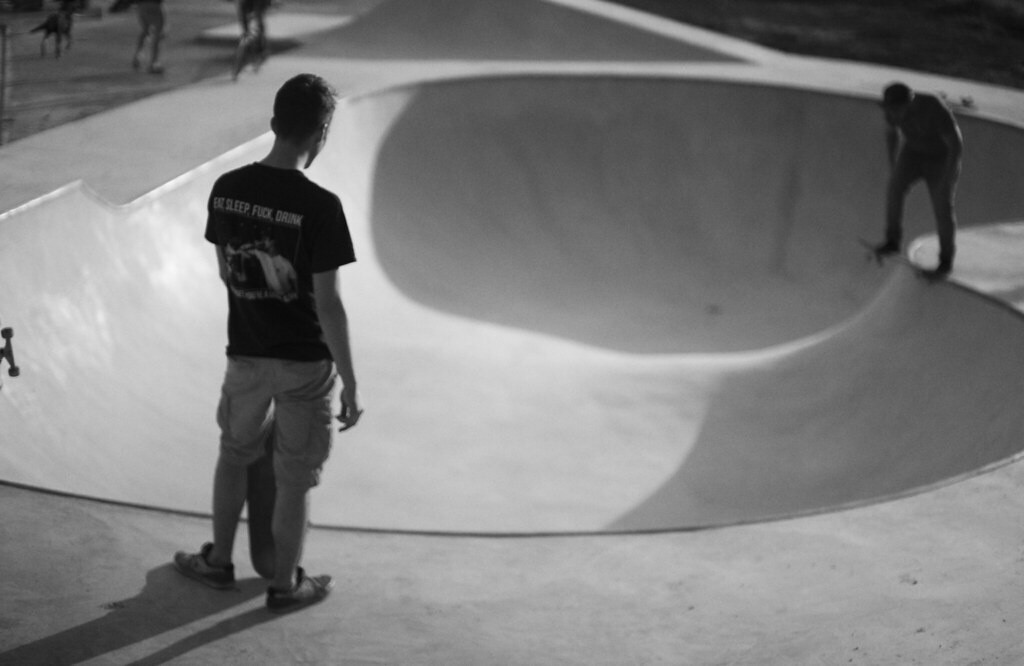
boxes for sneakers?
[171,541,236,589]
[266,566,336,613]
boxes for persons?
[174,74,360,614]
[239,0,271,52]
[876,83,964,279]
[111,0,166,73]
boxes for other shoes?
[937,260,954,275]
[878,239,898,254]
